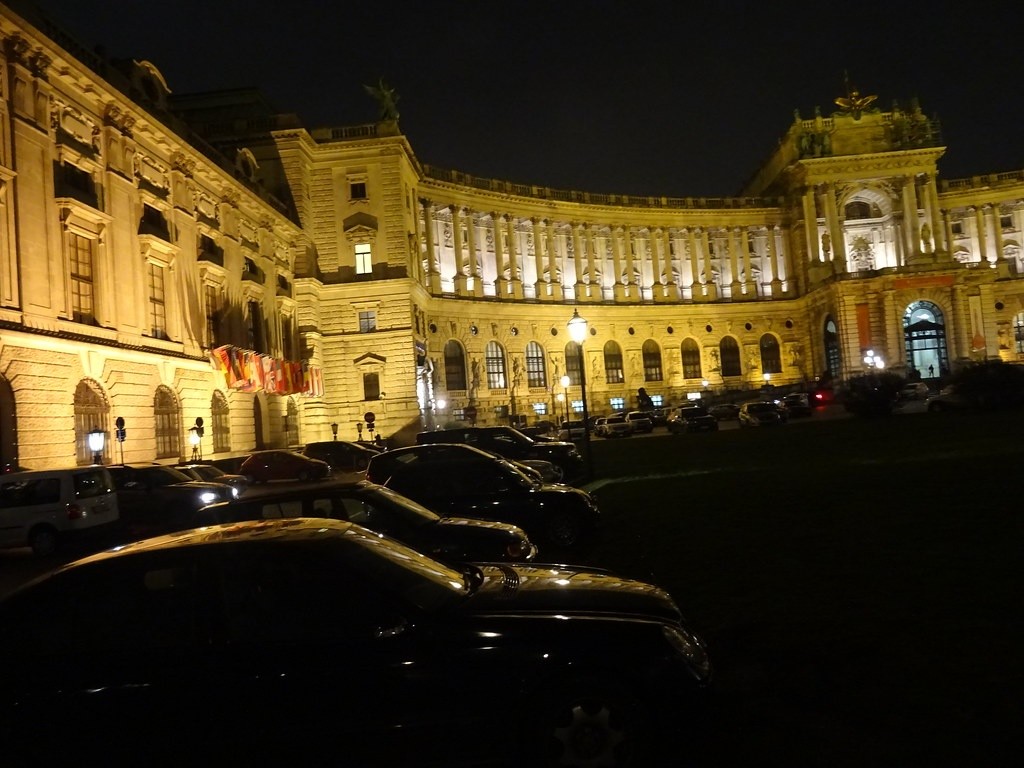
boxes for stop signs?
[364,412,375,422]
[467,407,477,418]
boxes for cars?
[783,391,812,418]
[666,408,676,431]
[901,382,930,400]
[583,415,606,430]
[925,382,992,411]
[0,518,709,768]
[626,410,654,433]
[240,449,331,486]
[558,420,587,442]
[366,443,601,550]
[386,449,564,485]
[707,404,741,420]
[352,441,389,454]
[602,417,632,438]
[107,462,240,530]
[416,426,579,465]
[655,406,677,424]
[172,463,248,497]
[738,400,781,429]
[300,441,381,471]
[525,420,556,436]
[594,418,606,437]
[186,477,540,563]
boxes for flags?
[216,345,324,398]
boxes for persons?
[822,231,830,251]
[929,365,934,377]
[747,348,758,368]
[789,346,801,366]
[591,356,604,378]
[668,351,680,374]
[509,356,527,389]
[630,354,643,377]
[471,355,486,388]
[548,355,566,386]
[921,223,931,244]
[709,351,720,370]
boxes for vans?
[0,464,122,560]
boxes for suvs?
[671,407,718,435]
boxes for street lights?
[188,429,201,464]
[88,425,105,464]
[863,349,884,374]
[566,307,592,443]
[560,376,573,444]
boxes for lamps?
[357,423,363,433]
[87,425,106,452]
[188,427,201,445]
[331,422,338,434]
